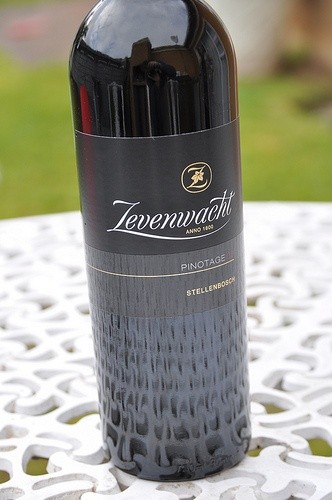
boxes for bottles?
[68,0,251,481]
